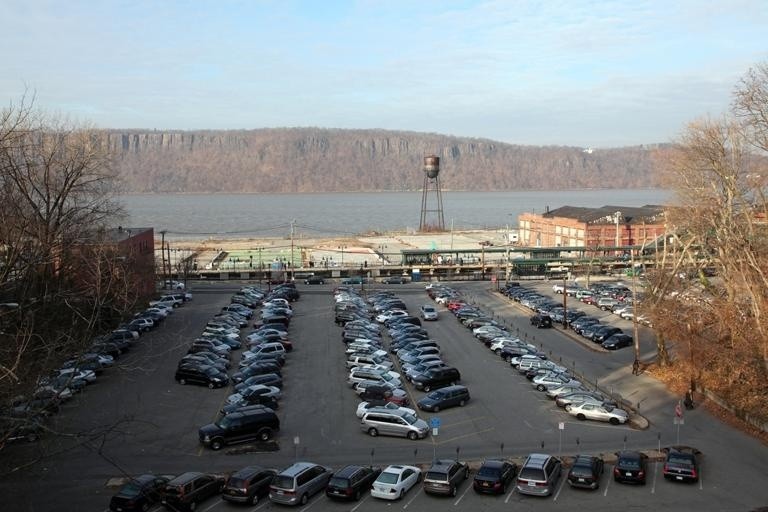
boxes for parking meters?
[368,447,375,466]
[574,436,579,453]
[622,436,627,451]
[412,449,417,467]
[455,446,459,462]
[657,431,660,455]
[539,440,544,453]
[499,442,504,461]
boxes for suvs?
[161,470,226,511]
[425,459,470,496]
[223,464,285,507]
[325,464,381,502]
[515,452,561,497]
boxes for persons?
[684,388,694,410]
[632,360,640,376]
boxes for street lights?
[213,247,222,267]
[504,247,514,265]
[228,255,239,275]
[337,244,347,267]
[255,246,265,289]
[615,211,622,256]
[171,248,179,268]
[377,241,387,267]
[296,246,306,268]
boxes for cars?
[472,459,517,496]
[1,292,191,446]
[175,285,301,448]
[160,279,184,289]
[479,240,492,247]
[567,454,604,493]
[108,474,177,511]
[611,449,648,485]
[302,276,323,285]
[381,275,407,284]
[663,450,698,482]
[370,464,422,500]
[333,285,471,440]
[266,277,290,285]
[342,274,362,285]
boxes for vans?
[269,461,332,506]
